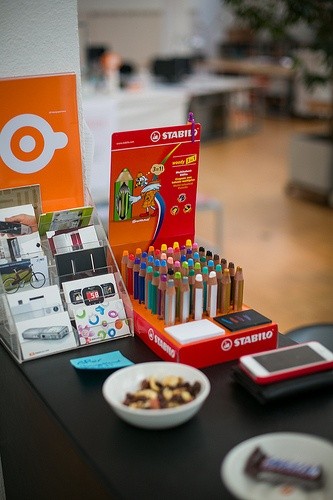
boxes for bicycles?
[3,263,46,295]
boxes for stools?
[284,324,333,353]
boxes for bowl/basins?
[101,361,211,430]
[220,432,333,500]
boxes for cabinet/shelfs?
[0,186,133,366]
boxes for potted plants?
[223,0,333,209]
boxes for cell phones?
[239,339,333,385]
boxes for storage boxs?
[120,289,279,368]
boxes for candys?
[242,447,326,490]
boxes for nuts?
[125,376,205,411]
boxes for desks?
[82,87,193,208]
[162,74,260,137]
[204,58,295,117]
[0,334,333,500]
